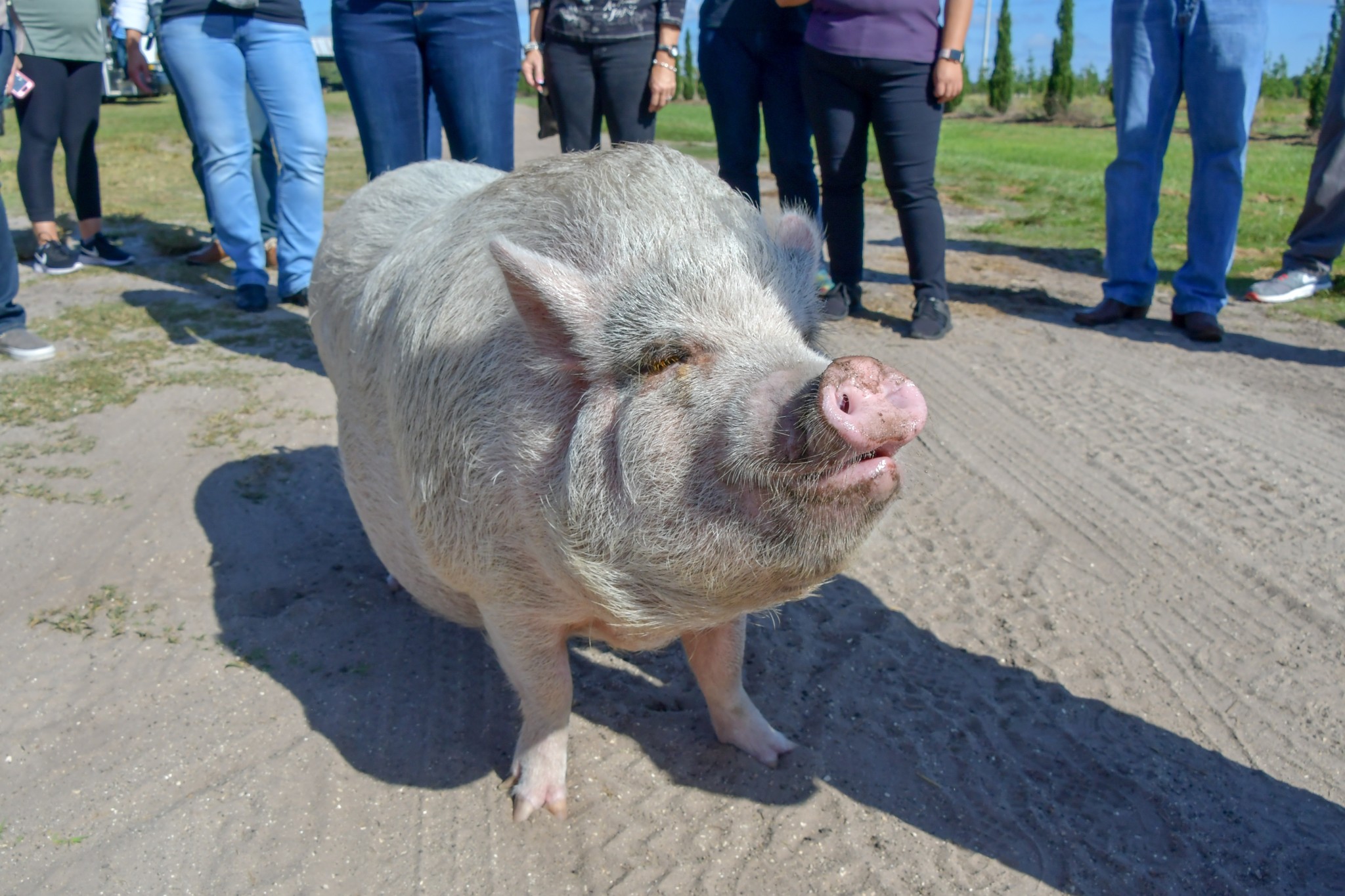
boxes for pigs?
[308,141,928,822]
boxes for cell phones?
[10,69,35,99]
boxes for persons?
[1074,0,1263,343]
[781,1,969,341]
[679,0,835,297]
[521,0,679,153]
[1244,22,1345,303]
[1,0,328,361]
[330,0,516,181]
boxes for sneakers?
[1171,310,1222,342]
[0,327,55,362]
[1073,298,1147,324]
[909,296,953,339]
[78,232,135,266]
[32,234,83,275]
[823,282,862,320]
[1245,265,1332,303]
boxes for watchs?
[657,44,680,57]
[938,49,964,65]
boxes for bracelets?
[523,42,544,54]
[652,59,677,73]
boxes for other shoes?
[186,239,231,265]
[235,284,268,312]
[267,247,280,270]
[283,287,307,306]
